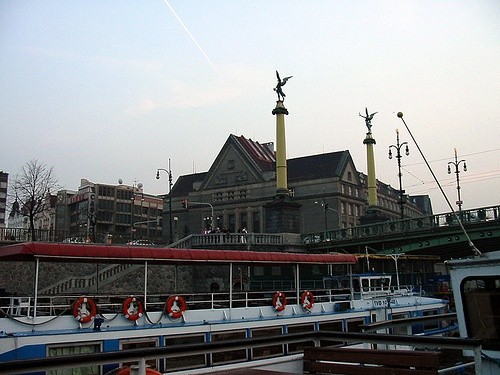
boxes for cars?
[126,239,154,246]
[62,237,92,244]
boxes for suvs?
[303,235,334,245]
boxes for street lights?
[388,127,410,219]
[155,159,173,245]
[447,148,467,223]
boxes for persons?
[70,236,91,244]
[275,79,286,104]
[365,115,372,134]
[207,227,231,243]
[238,227,248,243]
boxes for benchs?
[0,292,203,315]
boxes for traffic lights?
[182,199,187,209]
[325,203,329,212]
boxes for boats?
[0,241,460,375]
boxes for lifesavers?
[117,368,164,375]
[165,295,186,319]
[273,292,287,311]
[301,292,314,309]
[73,298,96,322]
[123,297,143,320]
